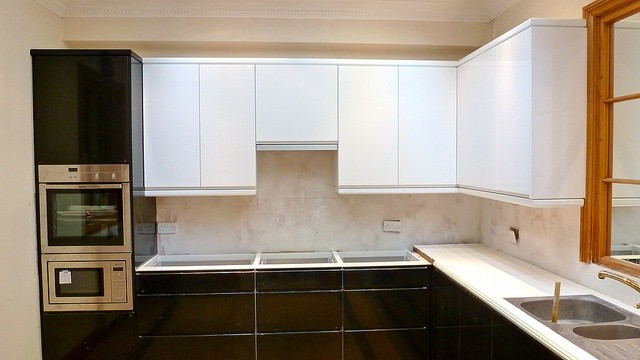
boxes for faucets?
[598,271,640,310]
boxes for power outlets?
[167,223,177,234]
[383,220,401,233]
[158,223,167,234]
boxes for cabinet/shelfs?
[455,18,587,209]
[610,22,640,209]
[141,57,256,197]
[256,59,337,151]
[338,60,459,196]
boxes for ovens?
[34,163,133,253]
[41,254,132,311]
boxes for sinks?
[544,322,640,360]
[504,294,640,322]
